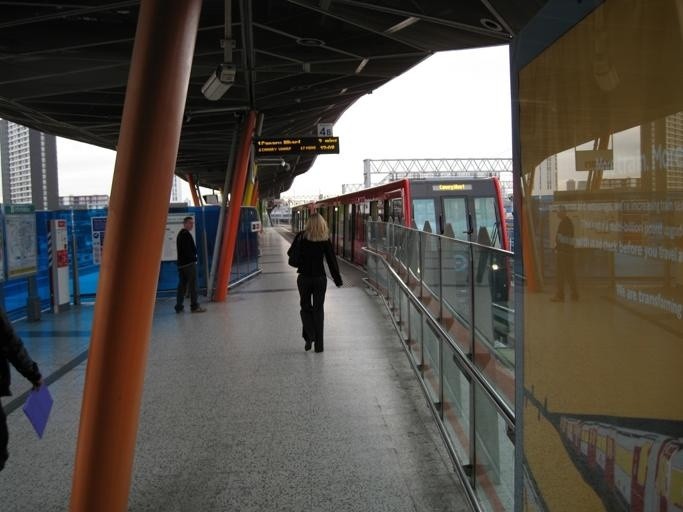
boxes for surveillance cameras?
[592,59,624,91]
[201,62,235,103]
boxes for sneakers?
[176,308,184,313]
[305,338,312,351]
[192,307,206,313]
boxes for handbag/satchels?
[289,245,302,268]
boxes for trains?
[289,174,510,359]
[555,412,682,512]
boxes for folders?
[23,383,53,439]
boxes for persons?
[283,211,342,355]
[171,216,207,313]
[474,249,511,349]
[0,307,41,467]
[548,204,584,304]
